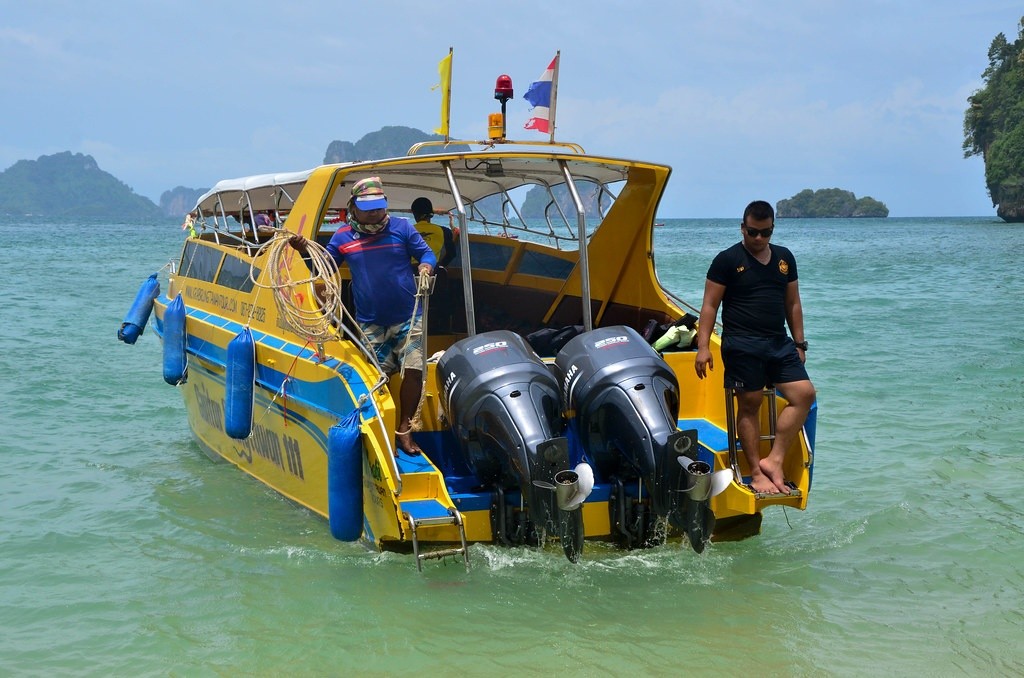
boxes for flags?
[522,54,558,133]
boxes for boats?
[152,42,821,572]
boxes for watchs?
[793,340,810,351]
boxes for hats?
[411,197,433,215]
[354,194,387,211]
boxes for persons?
[232,212,276,232]
[288,169,437,455]
[405,196,458,334]
[693,201,816,495]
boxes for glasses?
[743,223,774,238]
